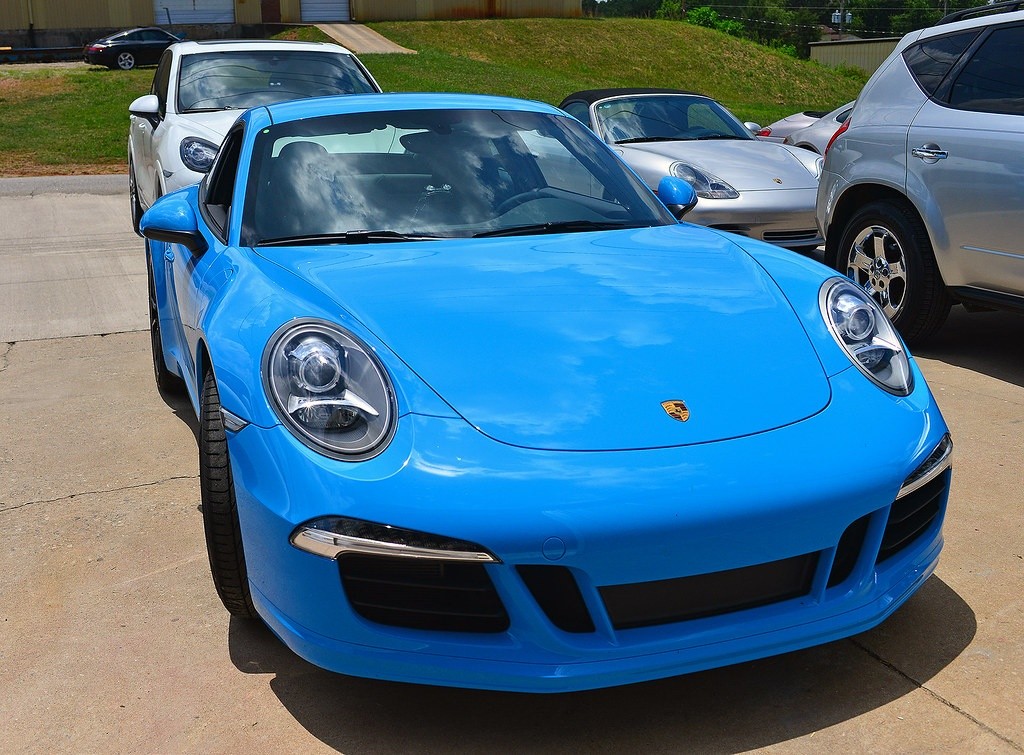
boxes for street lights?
[831,0,852,40]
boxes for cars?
[782,100,856,157]
[517,88,826,253]
[127,38,429,236]
[754,109,830,145]
[83,27,181,70]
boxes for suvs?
[813,4,1024,351]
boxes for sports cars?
[140,93,953,694]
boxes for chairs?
[260,141,366,243]
[414,130,508,235]
